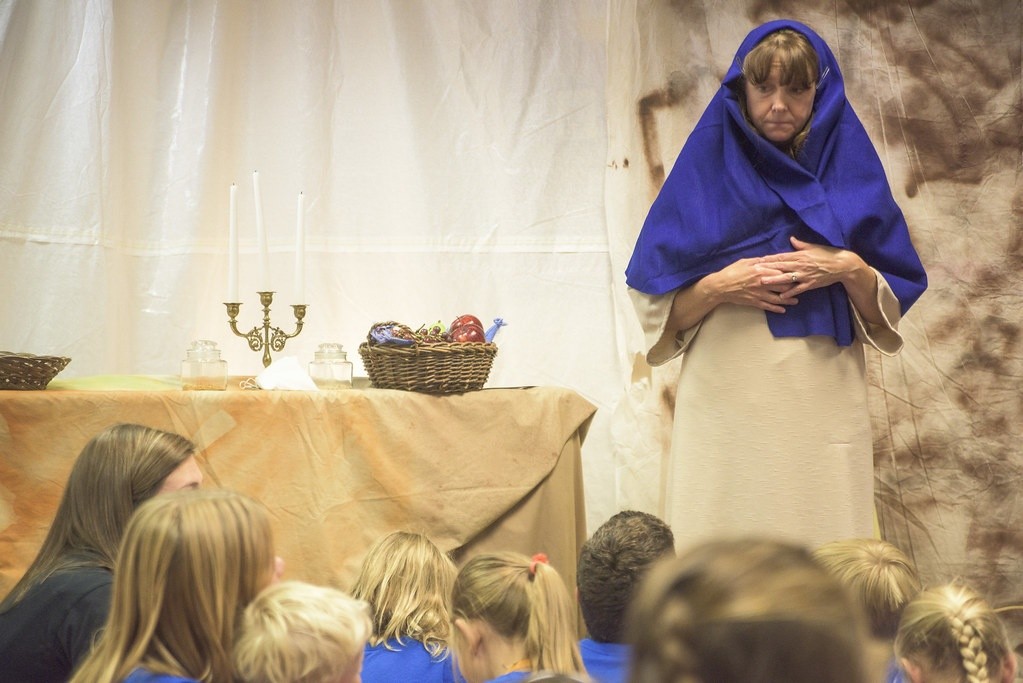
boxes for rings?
[791,271,797,282]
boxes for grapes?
[392,325,452,342]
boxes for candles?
[286,189,313,307]
[221,181,246,305]
[250,169,279,292]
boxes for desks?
[0,373,599,599]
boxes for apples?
[450,315,485,343]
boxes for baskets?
[0,351,72,390]
[358,321,498,393]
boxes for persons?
[814,539,920,681]
[895,577,1018,682]
[620,534,864,682]
[350,528,466,682]
[230,577,371,681]
[0,422,205,682]
[574,510,676,681]
[622,18,933,554]
[65,487,283,682]
[444,552,594,682]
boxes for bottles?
[310,343,353,390]
[179,340,228,391]
